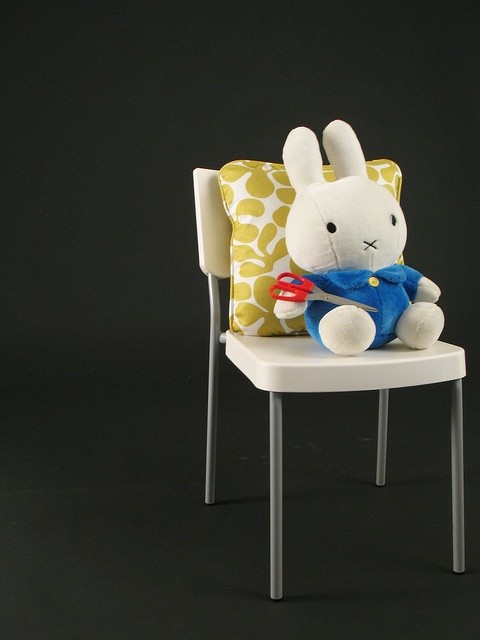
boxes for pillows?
[218,158,404,337]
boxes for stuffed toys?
[272,120,444,358]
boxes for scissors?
[269,272,380,312]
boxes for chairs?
[192,167,468,600]
